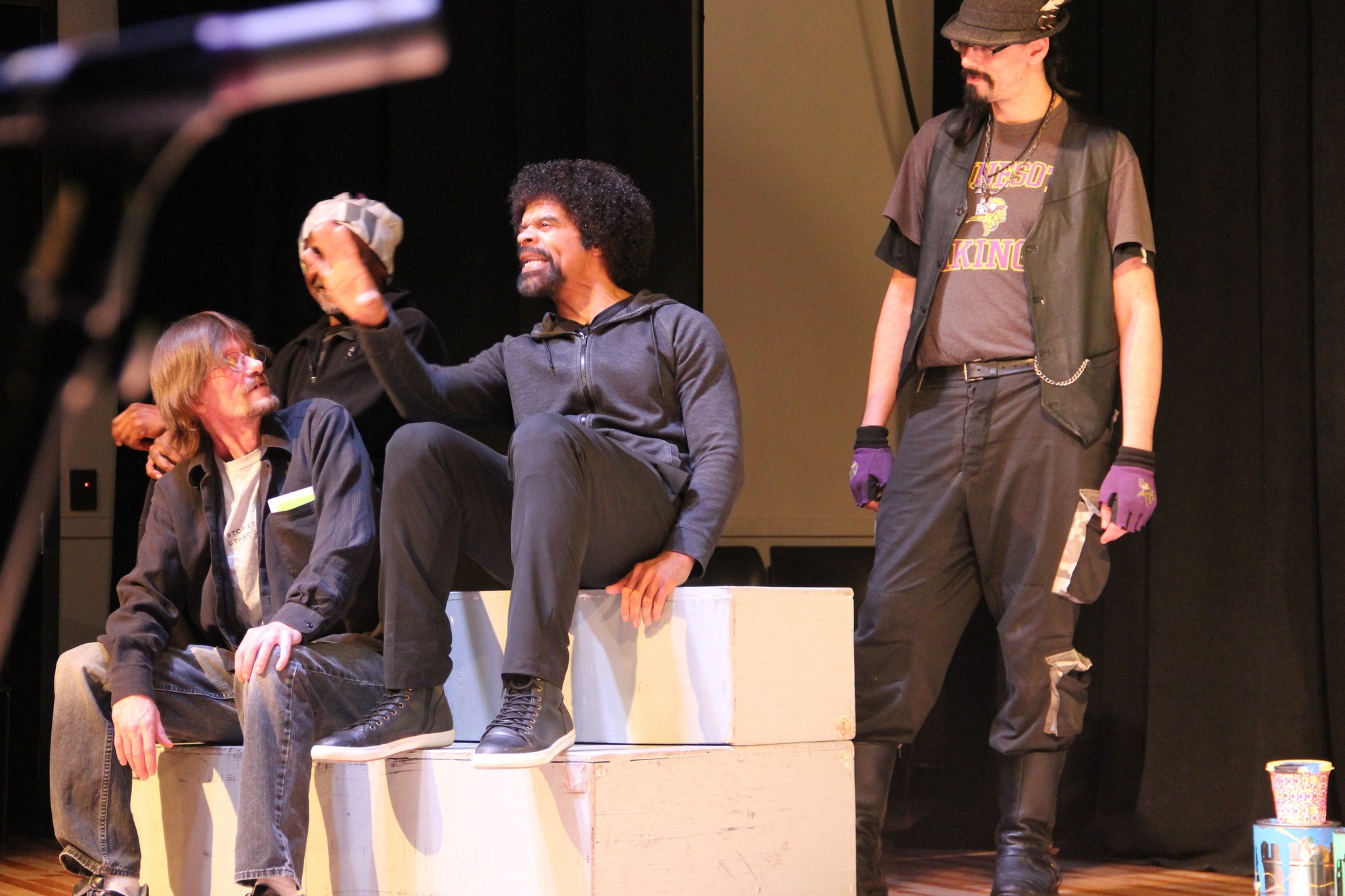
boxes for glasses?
[950,38,1010,59]
[205,348,267,372]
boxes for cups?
[1264,759,1335,827]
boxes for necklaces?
[974,91,1056,216]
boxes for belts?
[918,358,1040,381]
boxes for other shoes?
[245,884,306,896]
[71,874,149,896]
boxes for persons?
[112,193,451,650]
[49,312,391,896]
[847,1,1162,896]
[303,159,746,769]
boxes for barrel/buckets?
[1252,818,1345,896]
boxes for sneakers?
[473,671,576,768]
[310,682,456,763]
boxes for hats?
[941,0,1071,46]
[296,191,404,275]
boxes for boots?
[989,750,1062,896]
[853,742,899,896]
